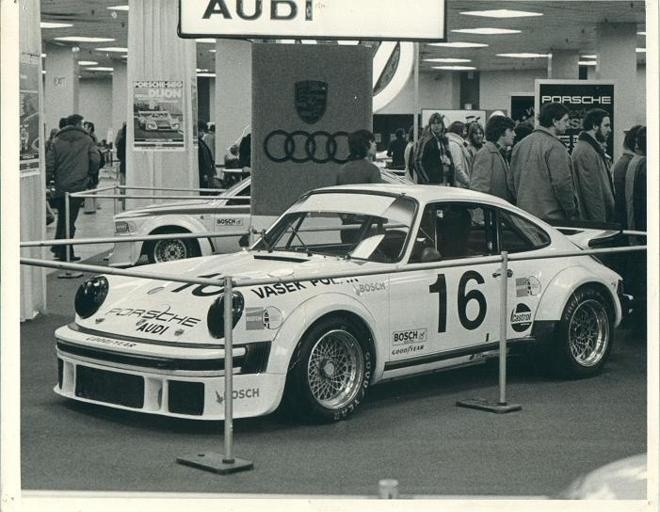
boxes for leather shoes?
[55,255,80,260]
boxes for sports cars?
[54,183,647,424]
[105,166,414,269]
[139,109,181,133]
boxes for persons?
[336,128,381,185]
[388,104,647,331]
[45,114,102,261]
[45,114,127,224]
[197,118,251,196]
